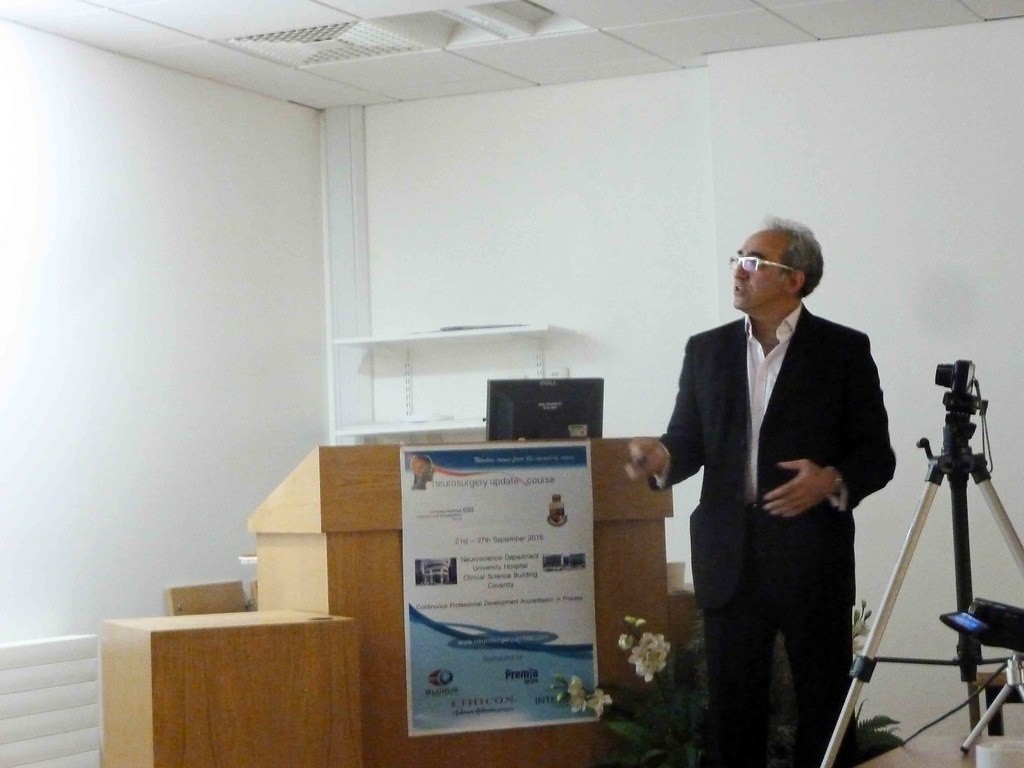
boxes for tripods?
[960,654,1024,754]
[821,389,1024,768]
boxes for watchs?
[826,466,844,497]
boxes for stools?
[976,672,1024,736]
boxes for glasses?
[728,256,793,272]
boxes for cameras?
[938,597,1024,654]
[936,358,976,393]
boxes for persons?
[624,221,896,768]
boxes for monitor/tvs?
[486,378,604,439]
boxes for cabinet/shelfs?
[332,323,580,438]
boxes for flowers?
[551,598,905,768]
